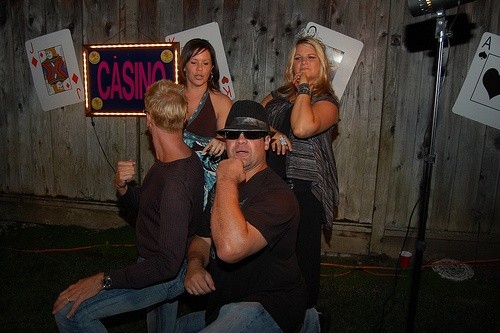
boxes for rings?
[66,296,71,303]
[279,136,287,145]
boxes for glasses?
[225,131,268,139]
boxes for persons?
[51,79,205,333]
[147,100,331,333]
[260,32,341,304]
[178,38,232,212]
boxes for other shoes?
[299,307,321,333]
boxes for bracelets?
[296,82,311,96]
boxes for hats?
[215,100,277,138]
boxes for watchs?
[100,271,112,289]
[111,180,127,190]
